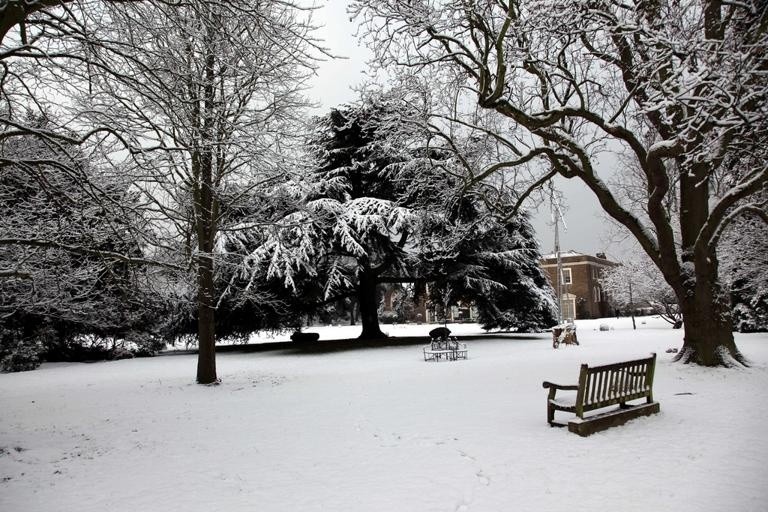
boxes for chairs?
[422,336,470,362]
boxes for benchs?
[543,353,663,436]
